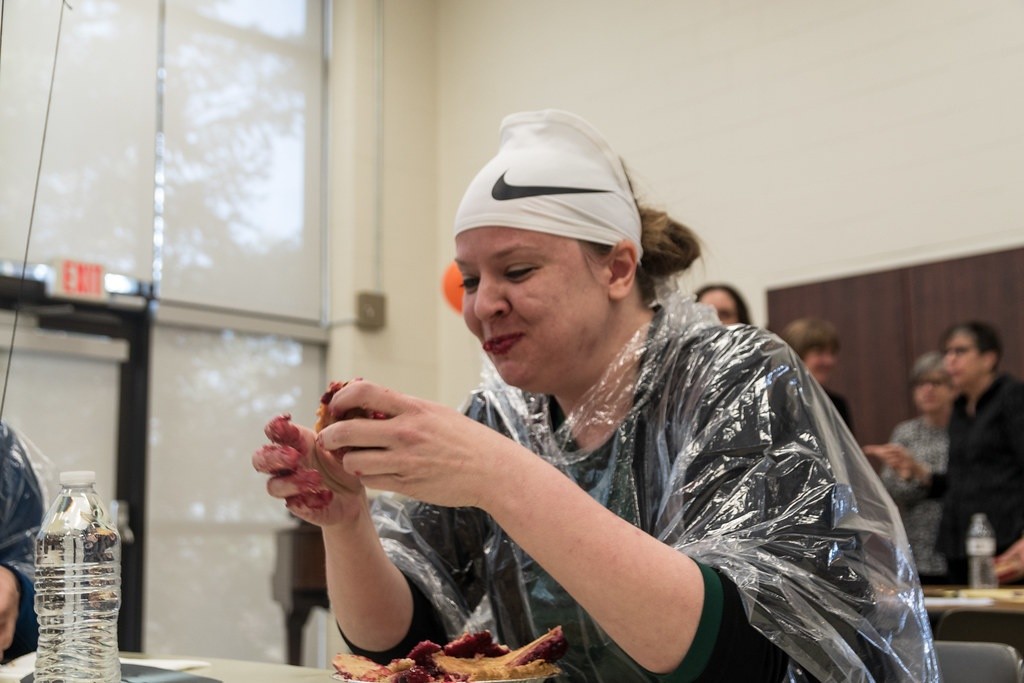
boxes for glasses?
[944,345,978,356]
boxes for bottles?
[34,471,122,683]
[967,513,1001,589]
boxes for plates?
[330,670,559,683]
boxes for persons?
[882,350,956,584]
[253,108,940,683]
[783,317,1024,589]
[693,284,752,327]
[0,420,44,669]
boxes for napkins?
[0,652,211,675]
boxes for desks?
[0,651,348,683]
[923,586,1024,613]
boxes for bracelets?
[920,462,933,483]
